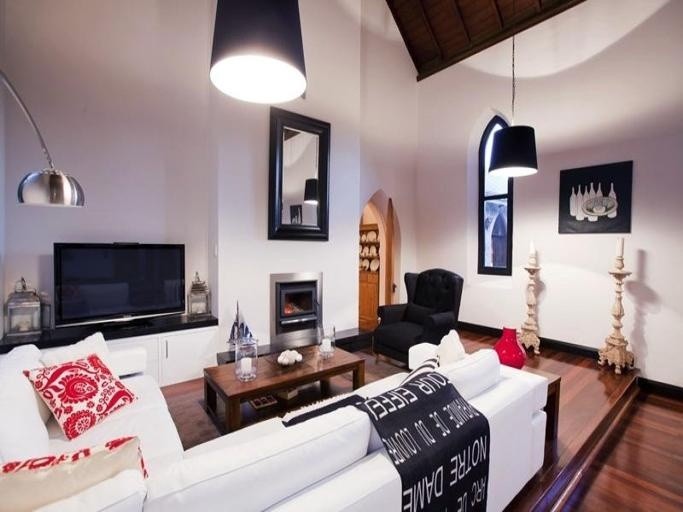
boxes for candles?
[19,321,30,330]
[241,359,252,376]
[531,248,536,256]
[322,339,331,352]
[196,308,204,313]
[618,238,624,257]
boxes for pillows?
[39,332,118,380]
[435,330,466,366]
[23,355,135,440]
[0,437,141,512]
[399,356,439,386]
[3,435,148,480]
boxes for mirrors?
[268,105,332,242]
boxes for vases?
[494,327,527,370]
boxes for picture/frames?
[290,205,303,226]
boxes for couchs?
[34,343,547,512]
[372,269,462,365]
[0,344,185,512]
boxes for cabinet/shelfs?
[0,312,218,388]
[359,224,379,332]
[217,328,374,365]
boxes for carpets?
[160,351,409,452]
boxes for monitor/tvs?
[53,242,187,332]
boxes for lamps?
[209,0,308,106]
[0,69,84,207]
[489,35,537,177]
[303,179,320,205]
